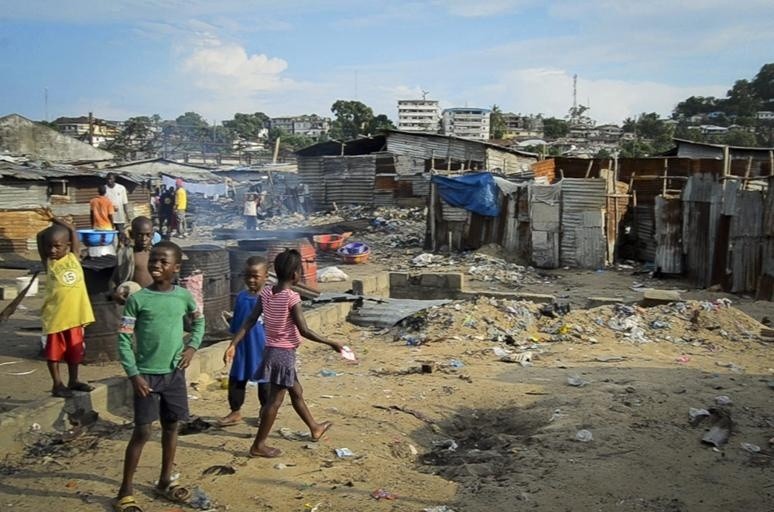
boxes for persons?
[216,255,271,424]
[110,242,205,512]
[243,191,261,230]
[90,186,113,230]
[103,173,131,241]
[151,177,188,238]
[37,203,95,398]
[104,216,154,304]
[224,249,346,456]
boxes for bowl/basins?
[78,229,119,247]
[313,234,344,250]
[338,243,371,263]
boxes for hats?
[176,179,184,187]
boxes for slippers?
[68,383,96,392]
[311,420,332,443]
[248,446,286,459]
[152,481,192,503]
[216,417,242,426]
[52,386,74,398]
[112,495,143,512]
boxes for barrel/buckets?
[17,276,38,296]
